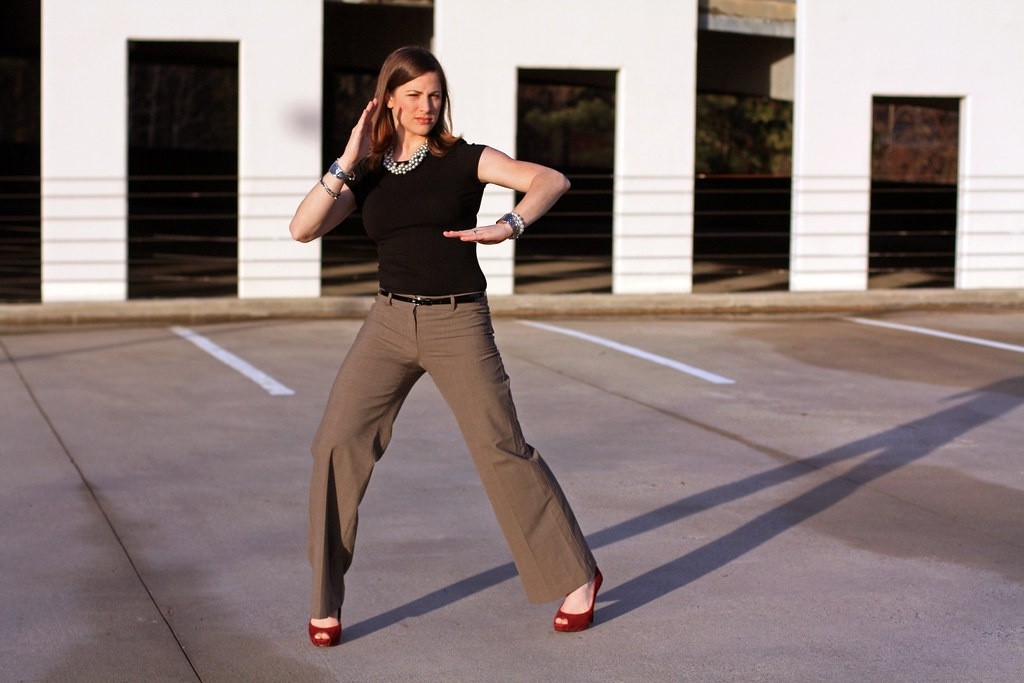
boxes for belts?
[379,288,484,305]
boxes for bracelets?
[320,177,341,200]
[330,160,356,181]
[497,211,524,240]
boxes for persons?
[289,45,606,646]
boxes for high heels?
[553,565,604,632]
[308,605,342,648]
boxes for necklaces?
[383,137,429,174]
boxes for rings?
[474,231,477,234]
